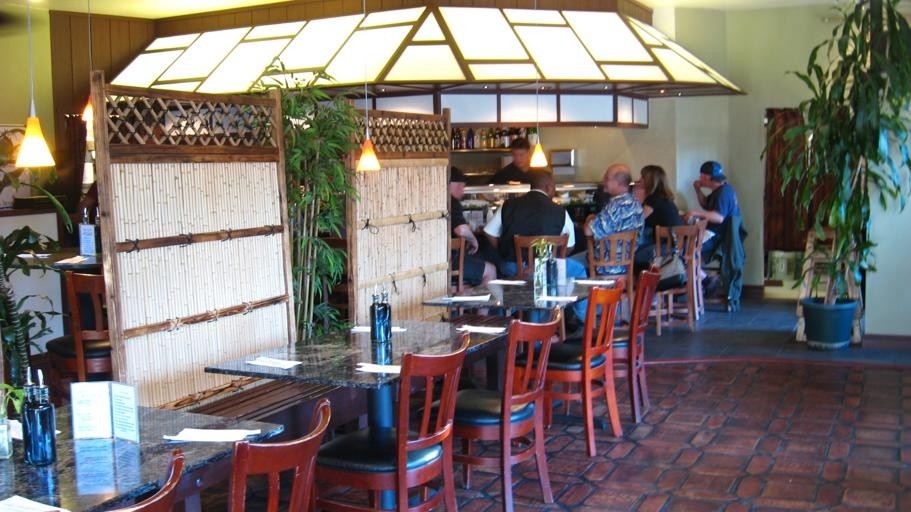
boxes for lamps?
[82,0,96,121]
[356,83,381,172]
[530,80,548,168]
[14,1,55,168]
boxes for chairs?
[417,304,561,512]
[512,278,624,457]
[98,447,186,511]
[451,238,466,295]
[662,220,709,321]
[46,272,114,410]
[513,234,570,280]
[562,263,661,422]
[229,398,332,511]
[314,329,472,512]
[636,225,696,336]
[586,231,637,329]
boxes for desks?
[449,181,598,243]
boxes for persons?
[634,165,686,264]
[682,161,742,297]
[482,170,597,343]
[568,163,645,340]
[490,137,541,187]
[66,181,99,235]
[447,165,497,316]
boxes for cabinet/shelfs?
[448,125,540,186]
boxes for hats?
[700,161,726,181]
[451,167,467,182]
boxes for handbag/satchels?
[649,225,687,291]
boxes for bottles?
[502,130,510,148]
[488,132,494,148]
[32,386,56,466]
[461,129,467,149]
[454,128,461,149]
[474,129,481,149]
[381,293,392,340]
[22,384,38,464]
[547,252,557,284]
[466,128,475,149]
[481,128,488,149]
[494,127,502,148]
[370,292,384,343]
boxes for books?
[69,380,140,447]
[78,223,97,258]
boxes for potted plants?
[0,129,73,404]
[255,57,362,338]
[760,0,911,349]
[0,383,25,459]
[531,239,559,310]
